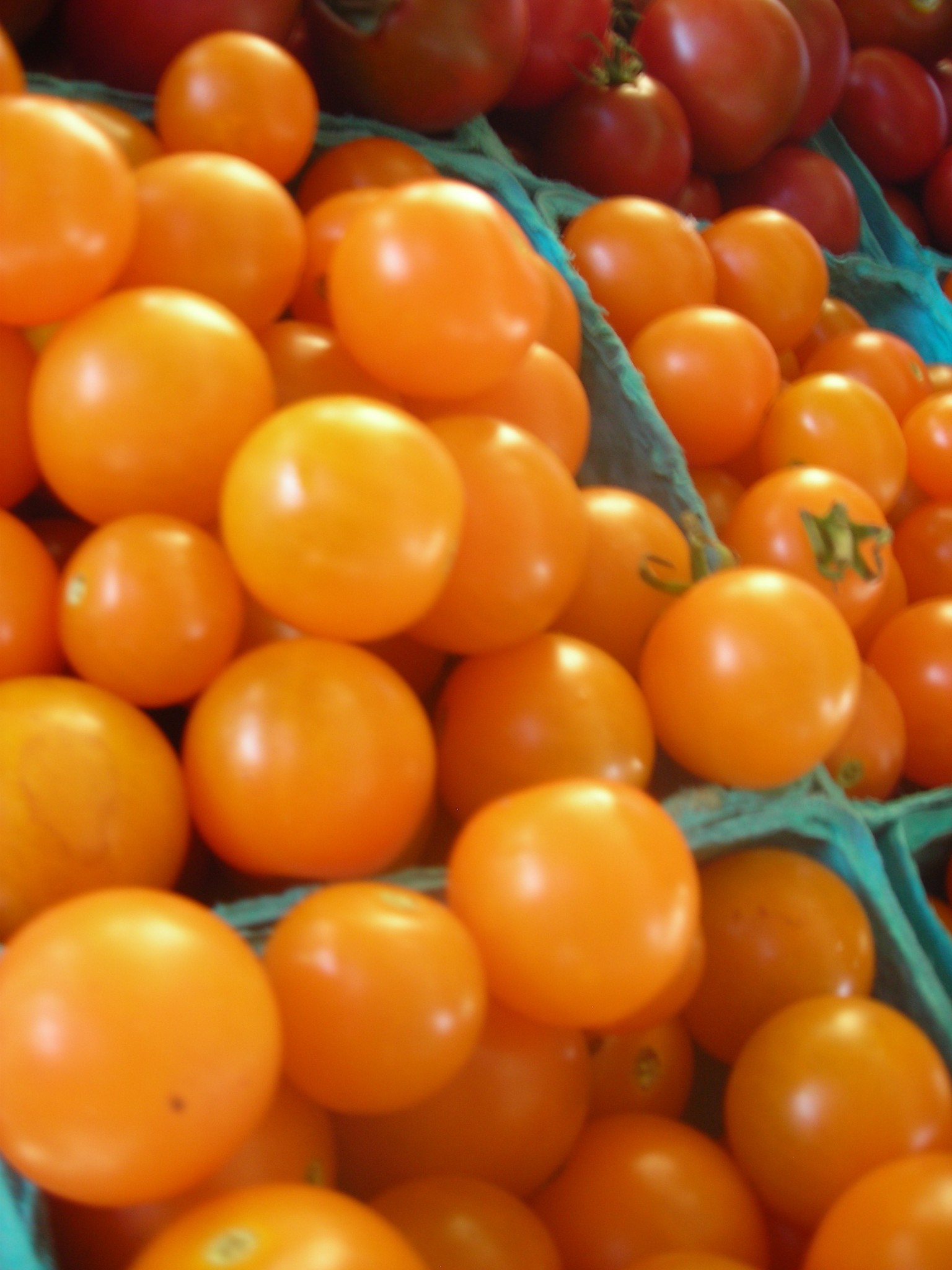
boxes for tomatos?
[0,30,952,1270]
[0,0,952,302]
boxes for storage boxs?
[40,78,950,944]
